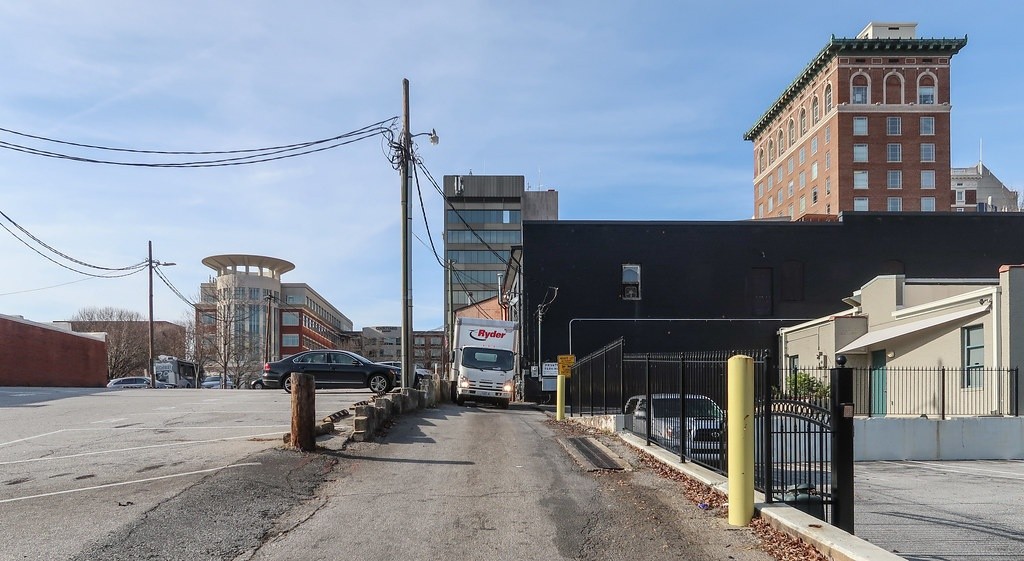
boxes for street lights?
[399,128,440,388]
[148,260,177,388]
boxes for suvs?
[625,393,727,462]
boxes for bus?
[153,355,205,389]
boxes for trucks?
[448,316,519,409]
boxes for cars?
[261,348,402,393]
[201,376,234,390]
[376,361,434,389]
[106,376,179,389]
[250,378,276,389]
